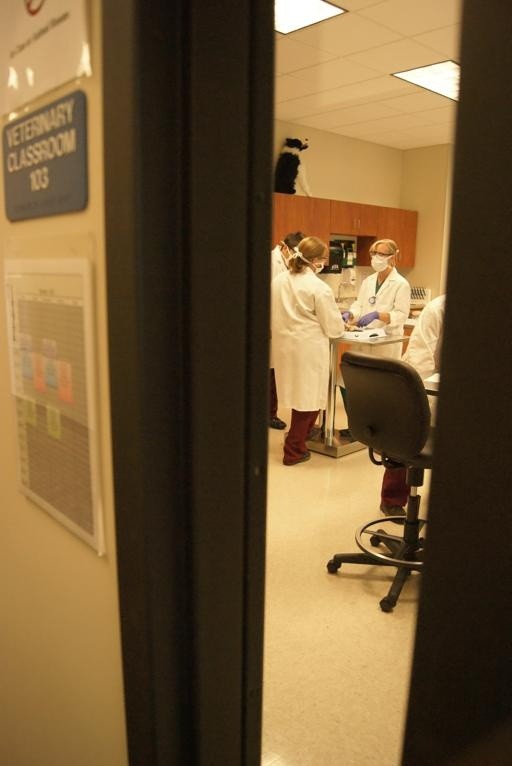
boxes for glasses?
[314,254,329,262]
[372,249,394,257]
[287,245,296,253]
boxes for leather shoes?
[269,418,287,429]
[380,506,407,523]
[286,452,311,465]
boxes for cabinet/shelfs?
[330,198,377,237]
[357,202,418,267]
[271,191,330,267]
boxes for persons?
[380,293,446,524]
[270,231,306,430]
[342,239,411,360]
[270,236,350,466]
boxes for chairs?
[327,350,435,614]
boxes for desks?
[285,332,410,459]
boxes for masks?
[293,248,324,273]
[370,253,395,271]
[280,242,296,267]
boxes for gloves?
[358,311,379,325]
[341,312,351,321]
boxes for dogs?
[275,137,313,198]
